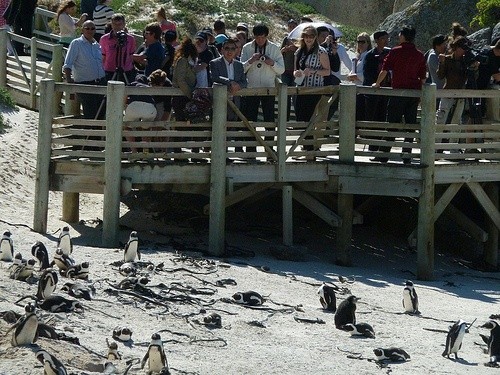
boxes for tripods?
[95,42,128,119]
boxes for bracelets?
[314,69,317,74]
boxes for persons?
[239,24,287,160]
[293,25,330,160]
[56,0,88,76]
[155,6,175,31]
[124,74,157,154]
[102,15,137,87]
[133,21,167,77]
[62,20,106,151]
[147,20,253,162]
[425,23,500,160]
[0,0,38,56]
[371,24,426,163]
[81,0,98,21]
[348,33,373,122]
[316,27,352,157]
[362,31,391,151]
[93,0,115,44]
[281,17,313,122]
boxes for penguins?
[200,311,222,328]
[114,277,152,289]
[316,280,376,339]
[231,290,267,306]
[107,341,124,360]
[0,226,93,313]
[34,349,68,375]
[371,346,411,364]
[401,279,422,315]
[477,320,500,366]
[440,318,471,361]
[123,230,141,262]
[119,262,137,277]
[113,324,133,342]
[4,301,63,347]
[140,331,171,375]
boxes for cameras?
[116,31,127,42]
[259,55,267,62]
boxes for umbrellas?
[287,20,343,41]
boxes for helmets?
[213,34,229,46]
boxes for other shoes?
[403,160,411,165]
[127,152,150,164]
[369,157,388,163]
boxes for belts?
[78,76,106,84]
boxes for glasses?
[82,27,97,30]
[223,47,237,51]
[459,45,468,50]
[302,33,316,39]
[357,40,367,44]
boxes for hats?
[194,31,209,42]
[237,22,249,29]
